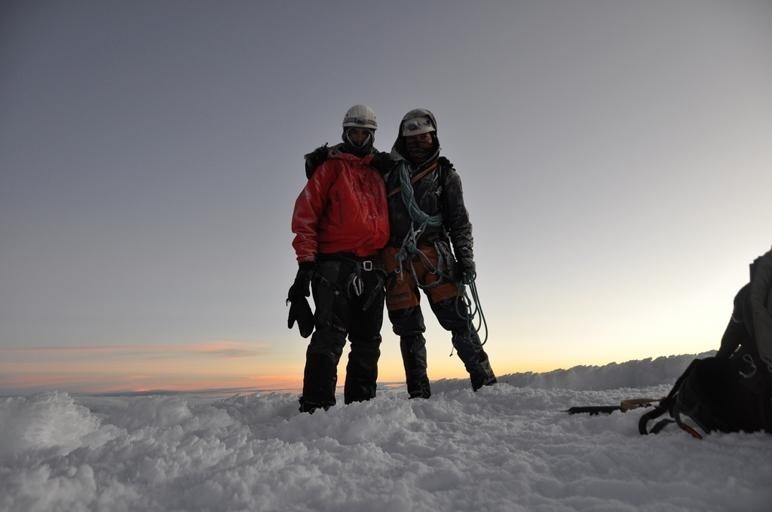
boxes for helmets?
[342,104,378,130]
[402,117,436,137]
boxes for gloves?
[287,261,314,337]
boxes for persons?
[291,104,390,414]
[715,248,772,359]
[385,108,497,398]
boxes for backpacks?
[669,247,771,433]
